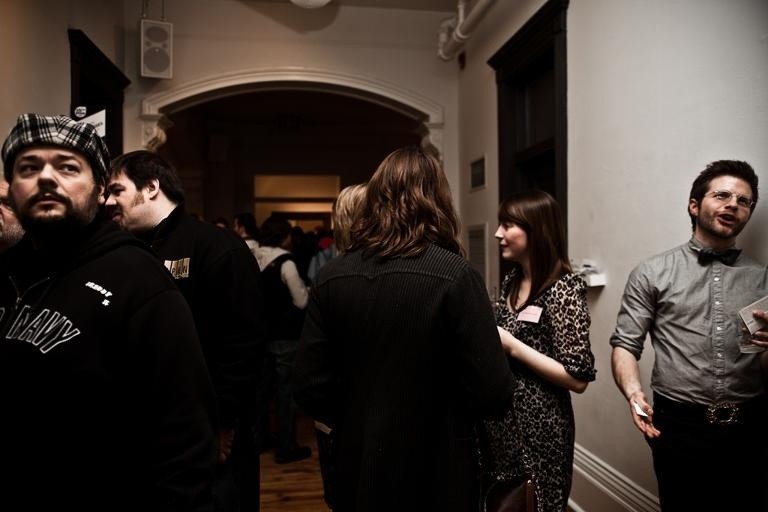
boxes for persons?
[0,113,198,511]
[214,216,337,463]
[0,174,26,256]
[608,158,767,511]
[475,188,597,511]
[302,144,517,511]
[307,181,371,510]
[100,149,276,511]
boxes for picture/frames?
[465,220,490,295]
[467,152,489,192]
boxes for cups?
[736,317,765,353]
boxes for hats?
[1,112,113,180]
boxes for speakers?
[137,18,175,80]
